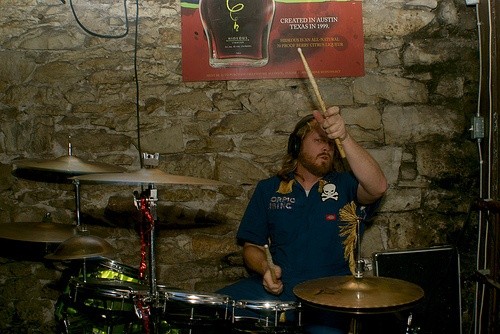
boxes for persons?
[211,106,388,334]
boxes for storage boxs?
[373,245,463,334]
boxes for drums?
[158,285,232,334]
[64,276,151,334]
[229,296,303,334]
[56,257,144,324]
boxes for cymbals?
[5,156,125,181]
[46,235,120,260]
[0,222,75,244]
[292,274,424,307]
[72,167,233,187]
